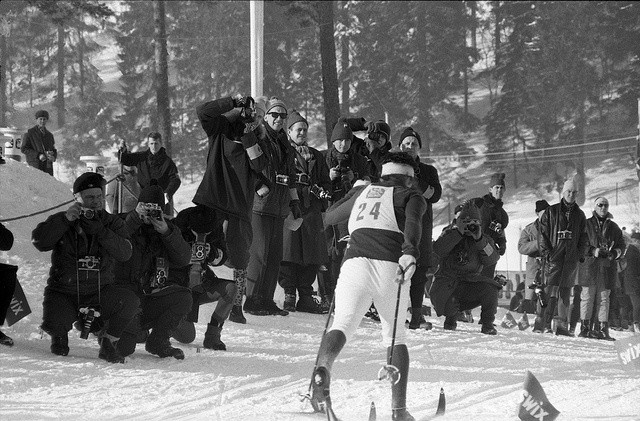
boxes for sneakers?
[99,338,124,363]
[146,336,184,359]
[312,367,331,412]
[263,298,289,315]
[243,298,269,315]
[321,293,329,313]
[393,409,415,421]
[50,336,68,355]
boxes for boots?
[581,320,600,338]
[556,319,574,337]
[204,323,227,350]
[229,305,246,323]
[296,287,322,313]
[284,288,296,312]
[569,322,576,333]
[545,322,553,334]
[599,322,616,341]
[532,316,544,333]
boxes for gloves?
[592,248,606,257]
[363,120,380,133]
[240,108,255,133]
[289,199,304,232]
[607,250,617,263]
[234,96,254,109]
[363,138,380,153]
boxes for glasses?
[597,203,608,208]
[268,112,288,119]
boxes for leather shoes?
[481,324,497,335]
[0,331,14,346]
[444,318,456,329]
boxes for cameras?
[239,102,257,126]
[464,217,480,235]
[296,170,312,187]
[77,255,103,272]
[368,127,381,143]
[140,202,166,220]
[80,308,102,340]
[43,150,55,158]
[454,245,472,265]
[150,257,171,289]
[495,273,511,294]
[313,184,325,201]
[191,232,210,262]
[600,243,616,261]
[491,221,504,234]
[276,172,292,189]
[73,204,100,222]
[333,152,352,184]
[529,281,547,307]
[556,230,573,240]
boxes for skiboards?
[325,386,446,420]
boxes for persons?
[351,118,393,178]
[310,151,426,421]
[610,226,630,328]
[532,177,587,337]
[0,223,19,346]
[577,197,626,341]
[469,173,509,325]
[192,93,270,323]
[624,232,640,334]
[317,116,379,313]
[32,172,141,363]
[243,95,301,314]
[425,200,502,335]
[442,204,467,322]
[518,199,552,333]
[399,127,442,330]
[118,132,181,214]
[21,110,57,175]
[277,106,332,314]
[117,179,192,359]
[171,202,238,350]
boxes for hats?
[594,197,608,208]
[254,96,269,116]
[563,177,578,193]
[368,120,390,140]
[536,200,549,211]
[331,116,353,141]
[399,127,421,149]
[460,200,480,220]
[73,172,103,193]
[262,96,287,114]
[489,173,505,188]
[287,106,308,129]
[139,178,165,204]
[36,110,48,120]
[186,203,217,233]
